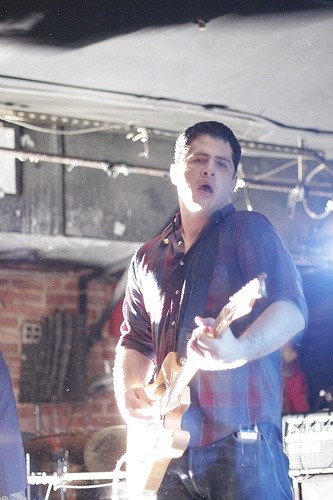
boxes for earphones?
[162,239,169,245]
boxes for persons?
[111,121,310,499]
[0,350,30,498]
[281,342,309,415]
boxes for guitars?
[122,272,269,493]
